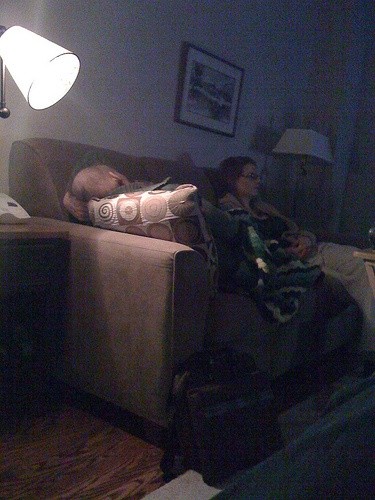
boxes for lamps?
[270,129,335,218]
[0,25,82,225]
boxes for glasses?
[238,172,262,181]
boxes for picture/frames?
[174,42,244,137]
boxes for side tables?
[0,224,72,409]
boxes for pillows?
[87,184,218,299]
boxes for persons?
[63,150,350,324]
[218,156,313,263]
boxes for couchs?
[9,138,375,475]
